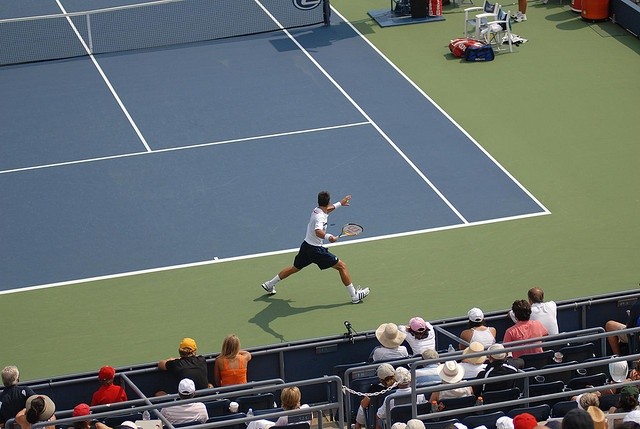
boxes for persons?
[245,385,314,429]
[0,365,30,418]
[526,288,560,334]
[628,360,640,381]
[599,394,622,414]
[155,337,214,396]
[260,191,371,304]
[94,421,129,429]
[617,420,640,429]
[455,341,490,378]
[160,377,211,428]
[403,316,439,358]
[360,363,396,409]
[477,343,519,399]
[389,420,408,429]
[213,335,252,388]
[428,360,477,404]
[512,412,539,429]
[91,365,127,406]
[606,354,633,383]
[505,300,546,356]
[14,394,57,429]
[414,348,443,377]
[561,407,594,429]
[443,422,468,429]
[619,384,639,412]
[495,415,516,429]
[72,402,91,428]
[372,322,409,363]
[375,365,428,428]
[462,308,497,351]
[605,296,640,354]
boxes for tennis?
[346,199,350,202]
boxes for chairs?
[436,395,478,416]
[515,350,555,369]
[566,372,607,391]
[592,395,624,409]
[558,343,595,363]
[206,412,246,423]
[204,399,231,415]
[582,355,619,374]
[462,410,504,429]
[477,5,513,54]
[525,380,565,402]
[268,422,311,429]
[235,393,275,411]
[508,404,550,427]
[481,387,520,411]
[103,413,147,427]
[542,361,578,378]
[324,362,366,399]
[252,407,285,418]
[421,417,459,429]
[551,400,578,418]
[464,0,498,40]
[390,402,432,424]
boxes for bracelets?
[324,233,333,240]
[332,201,342,210]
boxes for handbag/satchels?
[464,43,494,61]
[449,39,483,57]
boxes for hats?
[376,323,407,348]
[99,366,115,380]
[391,422,407,429]
[377,364,395,380]
[513,413,537,429]
[437,360,465,384]
[179,379,195,395]
[422,349,439,360]
[496,416,514,429]
[26,395,55,421]
[488,344,506,359]
[73,404,90,416]
[609,355,628,383]
[464,342,486,364]
[624,411,640,425]
[395,367,412,385]
[468,308,484,322]
[180,338,197,352]
[409,317,427,332]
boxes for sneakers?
[261,281,276,293]
[510,11,521,19]
[351,285,370,304]
[514,14,527,23]
